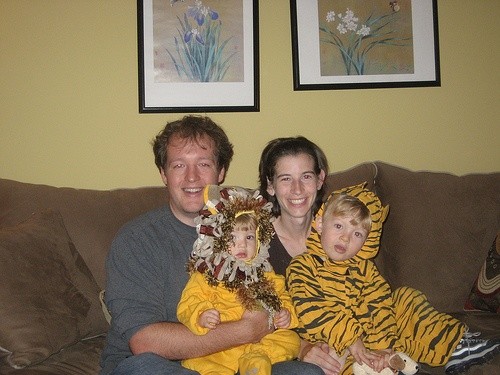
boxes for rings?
[372,362,373,365]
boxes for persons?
[285,182,500,375]
[101,114,324,375]
[176,184,301,375]
[254,136,392,375]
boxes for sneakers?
[444,331,500,375]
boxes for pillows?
[461,232,500,313]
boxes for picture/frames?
[288,0,441,91]
[138,0,261,114]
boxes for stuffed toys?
[352,349,419,375]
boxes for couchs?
[0,160,500,374]
